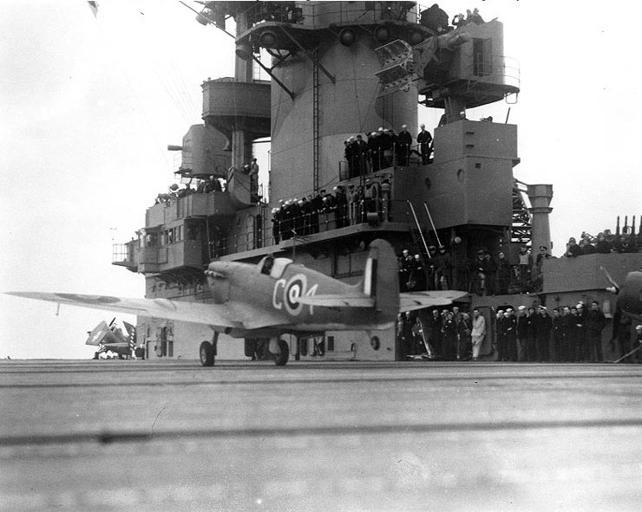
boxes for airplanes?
[597,261,642,364]
[82,315,139,363]
[0,233,471,373]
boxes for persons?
[396,229,640,364]
[272,123,432,244]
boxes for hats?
[395,299,592,318]
[402,245,549,262]
[270,178,373,217]
[344,124,425,148]
